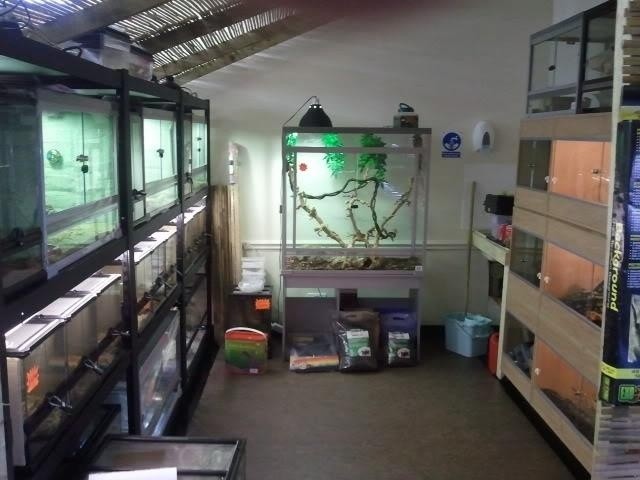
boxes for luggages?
[484,193,514,215]
[488,260,504,297]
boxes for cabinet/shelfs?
[525,0,618,111]
[1,72,220,480]
[496,112,613,475]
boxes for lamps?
[281,95,331,128]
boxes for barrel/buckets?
[223,326,268,375]
[488,332,498,376]
